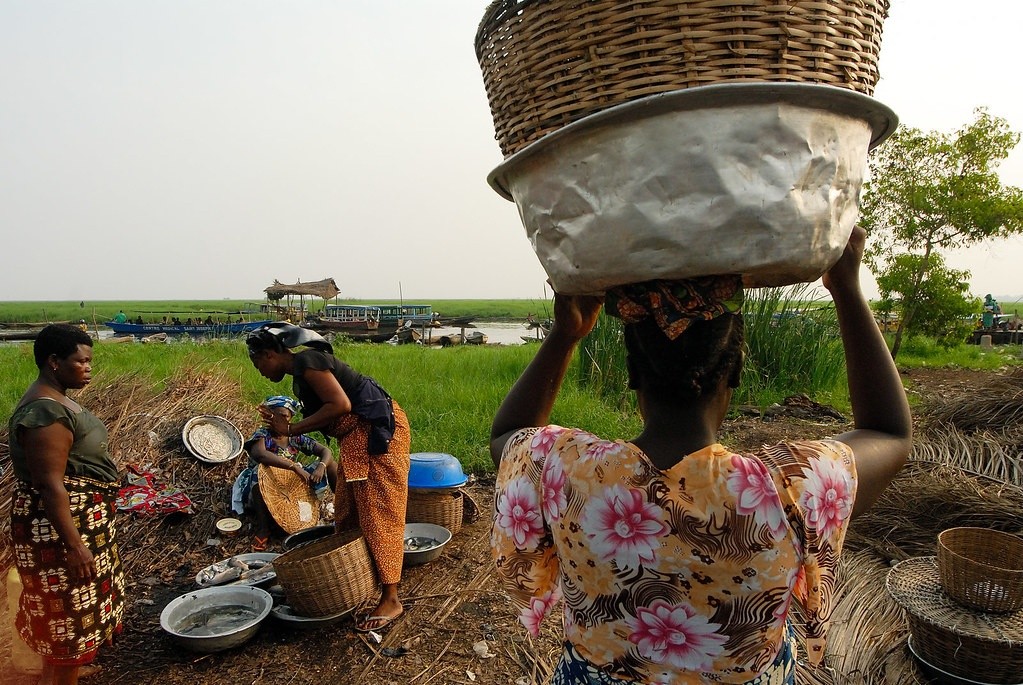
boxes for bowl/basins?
[403,522,452,562]
[488,80,900,295]
[216,518,242,535]
[182,415,244,463]
[408,452,469,487]
[160,585,273,649]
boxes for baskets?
[906,613,1023,685]
[936,526,1023,612]
[475,0,890,160]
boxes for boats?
[466,331,488,345]
[104,320,274,333]
[398,328,421,343]
[312,304,476,327]
[298,312,411,342]
[0,317,87,341]
[540,323,553,338]
[438,333,467,347]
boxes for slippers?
[354,604,404,632]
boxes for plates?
[195,552,283,586]
[273,600,357,626]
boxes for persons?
[489,223,914,684]
[135,316,144,324]
[225,316,231,324]
[243,395,339,551]
[8,326,126,685]
[79,319,87,333]
[111,310,133,324]
[160,316,181,325]
[982,294,1002,329]
[185,316,222,325]
[244,321,411,632]
[236,318,243,323]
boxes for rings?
[269,414,273,419]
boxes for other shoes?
[77,662,102,680]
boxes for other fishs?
[199,557,275,587]
[404,534,441,552]
[174,605,262,637]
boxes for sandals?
[253,527,269,550]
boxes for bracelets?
[287,423,292,436]
[289,464,300,470]
[320,462,326,467]
